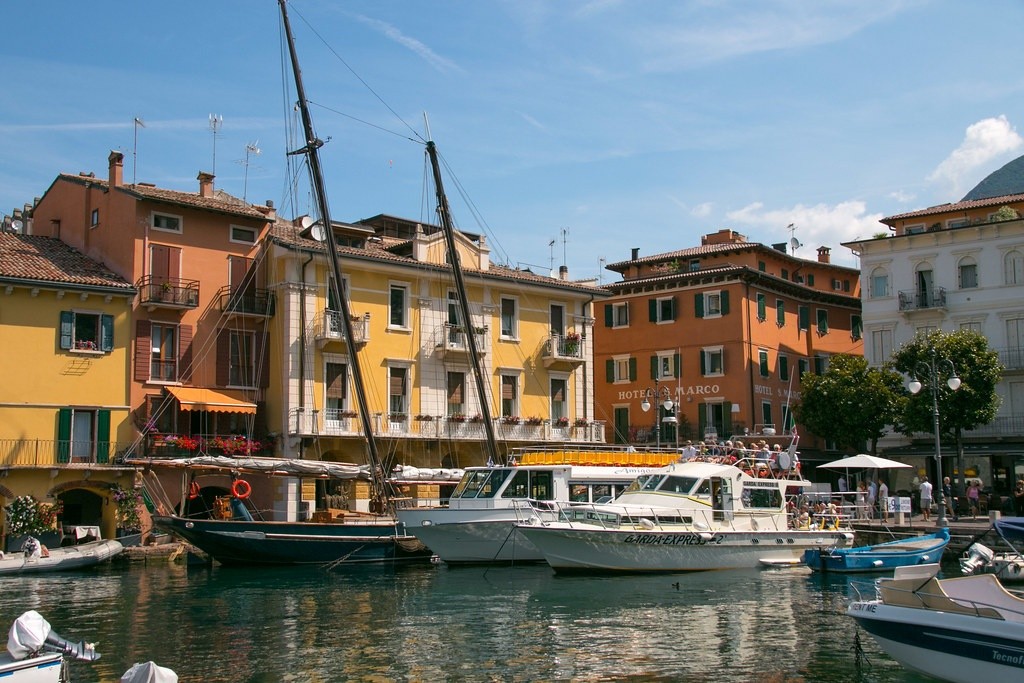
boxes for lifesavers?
[185,481,200,499]
[231,479,252,499]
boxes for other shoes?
[920,519,931,522]
[880,520,888,523]
[954,516,958,522]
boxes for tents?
[816,454,913,492]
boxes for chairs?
[977,494,1007,515]
[933,289,943,306]
[58,521,76,546]
[744,462,803,481]
[901,293,912,309]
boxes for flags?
[790,416,799,445]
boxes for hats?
[788,502,794,506]
[720,442,724,446]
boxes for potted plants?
[155,282,174,301]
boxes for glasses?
[801,507,804,509]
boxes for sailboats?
[114,1,638,577]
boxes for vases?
[144,421,275,456]
[117,528,142,545]
[5,533,62,552]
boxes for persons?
[681,440,798,478]
[1014,480,1024,517]
[919,476,932,521]
[838,474,847,492]
[966,479,982,521]
[856,476,878,519]
[789,501,837,530]
[879,478,889,523]
[943,477,958,521]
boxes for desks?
[63,526,101,545]
[986,496,1016,514]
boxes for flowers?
[343,410,590,427]
[1,494,65,538]
[651,258,682,275]
[110,487,146,533]
[565,330,581,353]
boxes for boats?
[758,558,805,569]
[0,537,126,577]
[804,525,952,577]
[845,512,1024,683]
[510,455,856,577]
[394,443,667,570]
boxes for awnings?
[164,385,257,414]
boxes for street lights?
[640,376,674,453]
[907,348,962,527]
